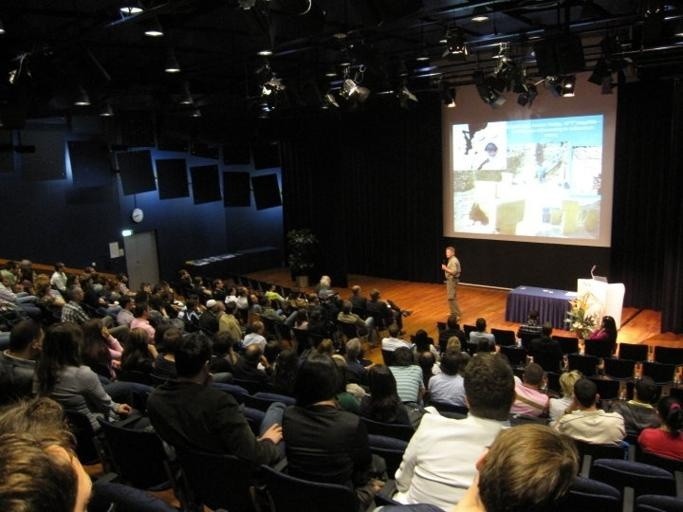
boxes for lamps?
[165,57,181,73]
[471,6,490,23]
[0,20,6,37]
[101,104,114,118]
[144,15,164,37]
[9,52,34,87]
[178,89,193,104]
[74,90,92,106]
[120,1,143,16]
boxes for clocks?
[130,208,144,223]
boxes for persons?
[29,322,140,435]
[361,363,417,443]
[0,396,181,512]
[414,312,682,463]
[368,424,579,512]
[0,250,154,331]
[282,352,389,504]
[441,246,462,316]
[335,366,362,413]
[146,332,287,486]
[472,142,506,170]
[378,350,517,511]
[0,319,47,404]
[154,266,414,359]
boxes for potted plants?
[284,228,319,286]
